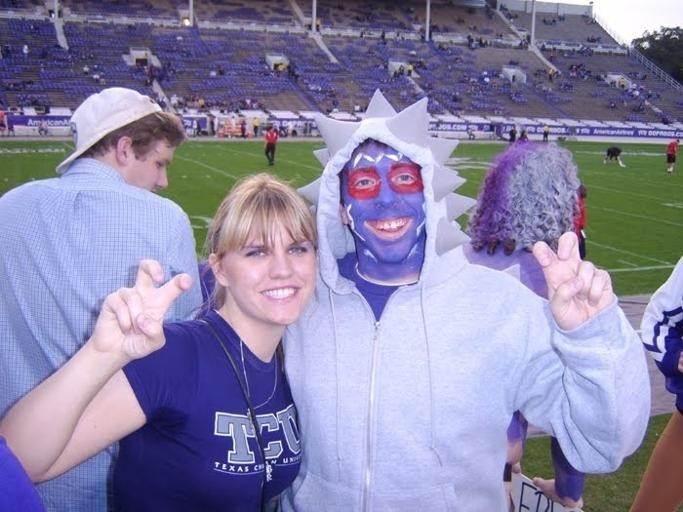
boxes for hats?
[55,86,163,173]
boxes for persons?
[628,256,682,512]
[470,139,587,508]
[1,86,203,512]
[2,172,317,511]
[280,88,651,512]
[2,0,683,174]
